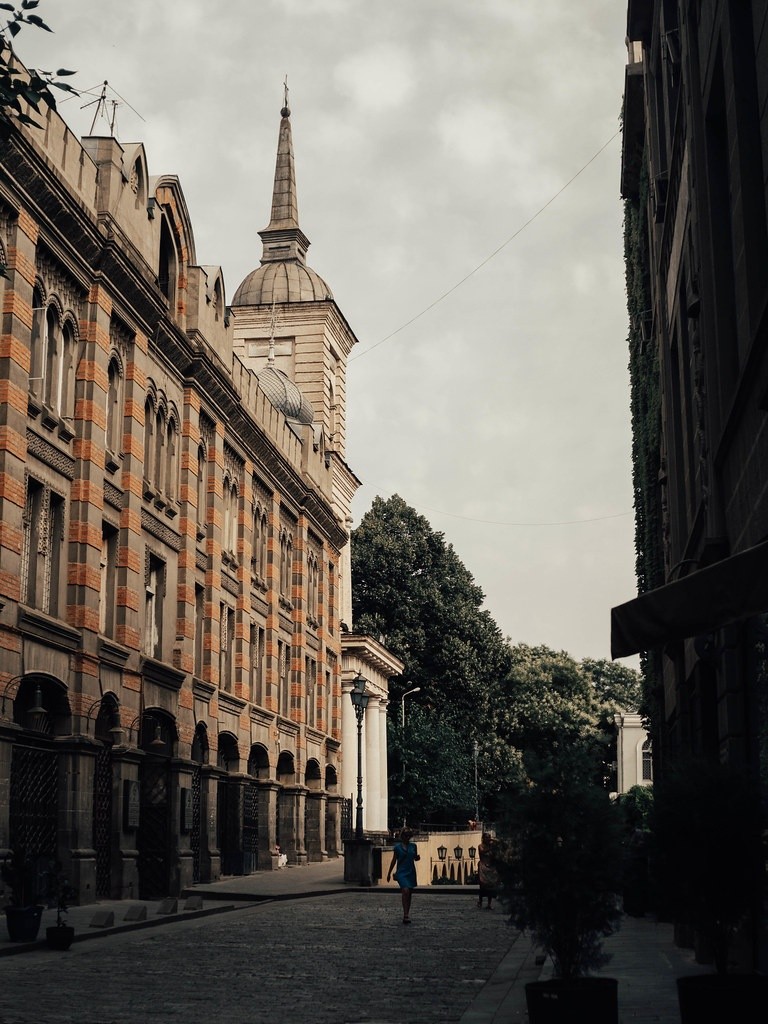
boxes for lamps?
[2,676,48,720]
[86,701,124,734]
[129,715,165,749]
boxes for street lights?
[350,670,376,839]
[471,738,482,824]
[400,685,421,826]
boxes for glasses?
[403,834,413,838]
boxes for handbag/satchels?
[393,873,398,880]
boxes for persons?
[475,833,497,911]
[387,828,421,923]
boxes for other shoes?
[403,916,411,923]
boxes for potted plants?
[46,884,80,951]
[643,745,767,1023]
[484,740,636,1024]
[0,842,52,942]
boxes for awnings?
[609,539,768,660]
